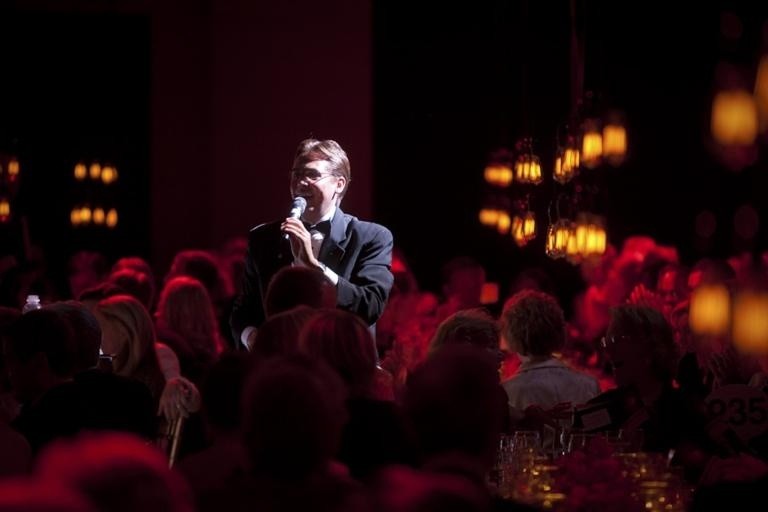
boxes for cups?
[498,430,689,510]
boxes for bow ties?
[302,220,332,233]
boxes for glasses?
[291,169,331,180]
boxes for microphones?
[282,197,307,240]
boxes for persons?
[0,137,768,512]
